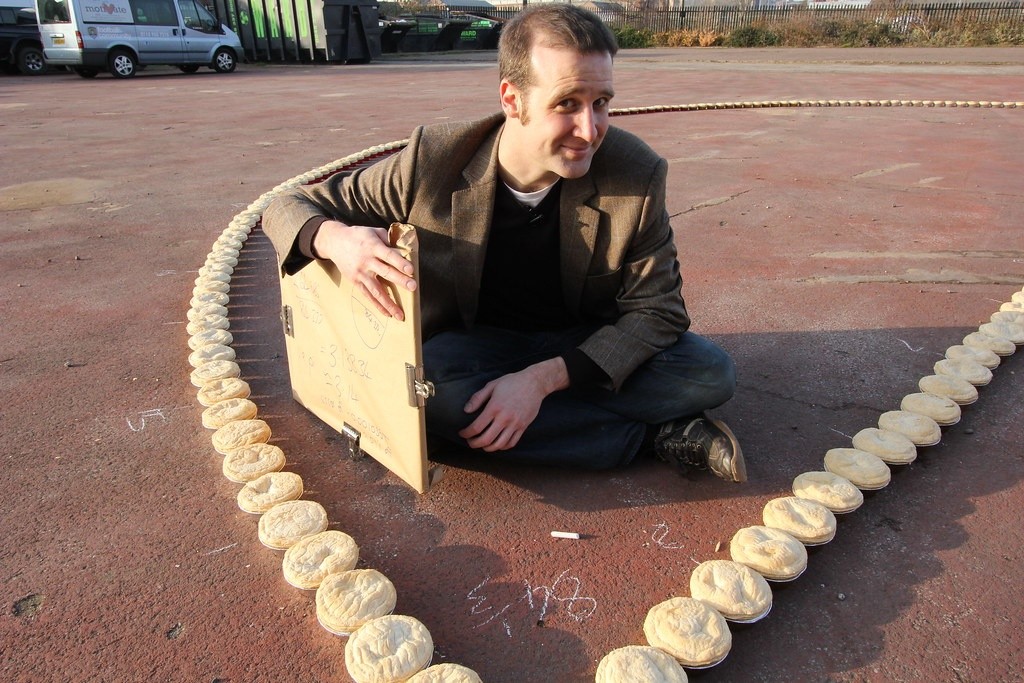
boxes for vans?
[34,0,245,78]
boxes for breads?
[608,100,1024,116]
[186,139,483,683]
[592,282,1024,683]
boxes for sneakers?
[653,409,748,485]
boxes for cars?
[0,6,46,77]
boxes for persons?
[261,3,749,485]
